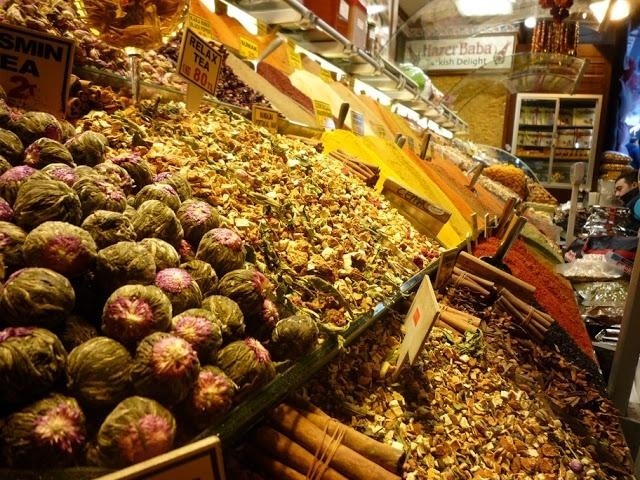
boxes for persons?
[614,171,640,223]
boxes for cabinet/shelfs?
[505,90,604,195]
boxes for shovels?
[479,215,527,276]
[242,37,283,73]
